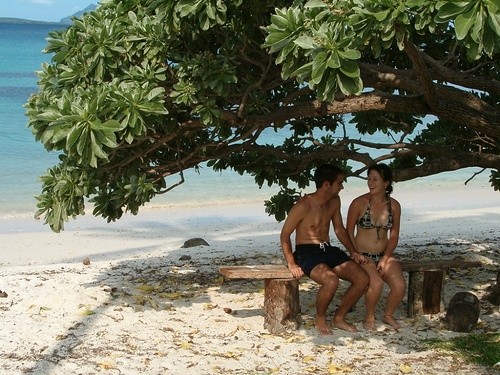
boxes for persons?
[346,163,406,330]
[280,164,368,335]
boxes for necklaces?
[314,191,326,210]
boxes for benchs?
[220,260,482,327]
